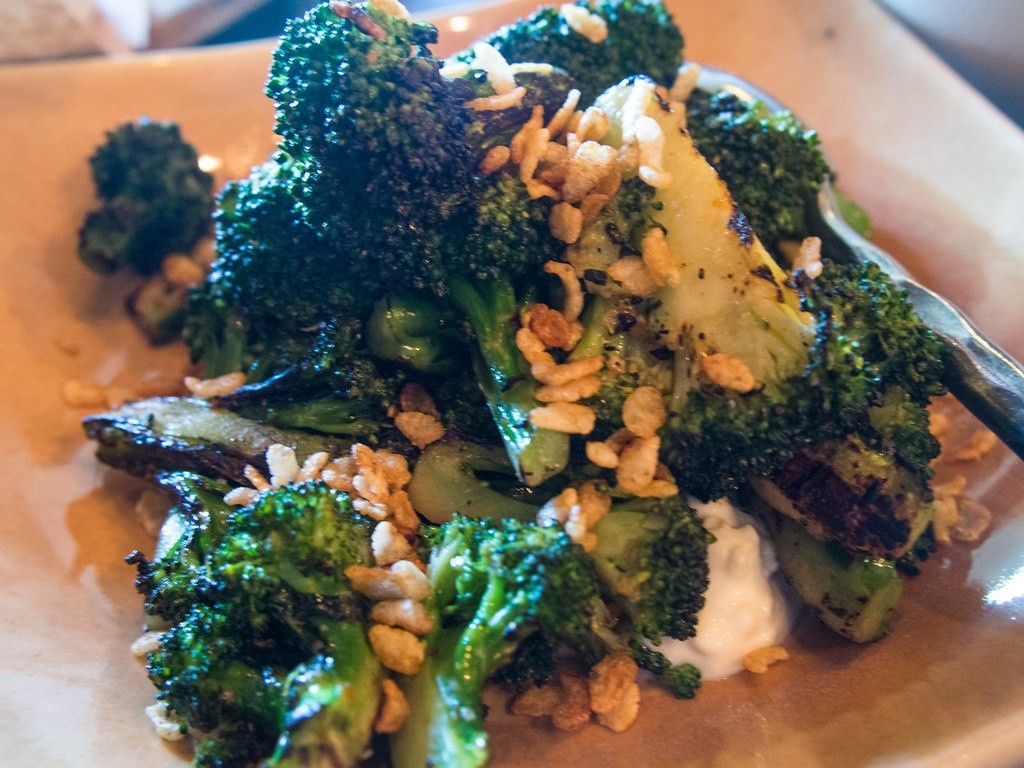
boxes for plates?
[0,2,1024,768]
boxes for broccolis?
[74,0,958,768]
